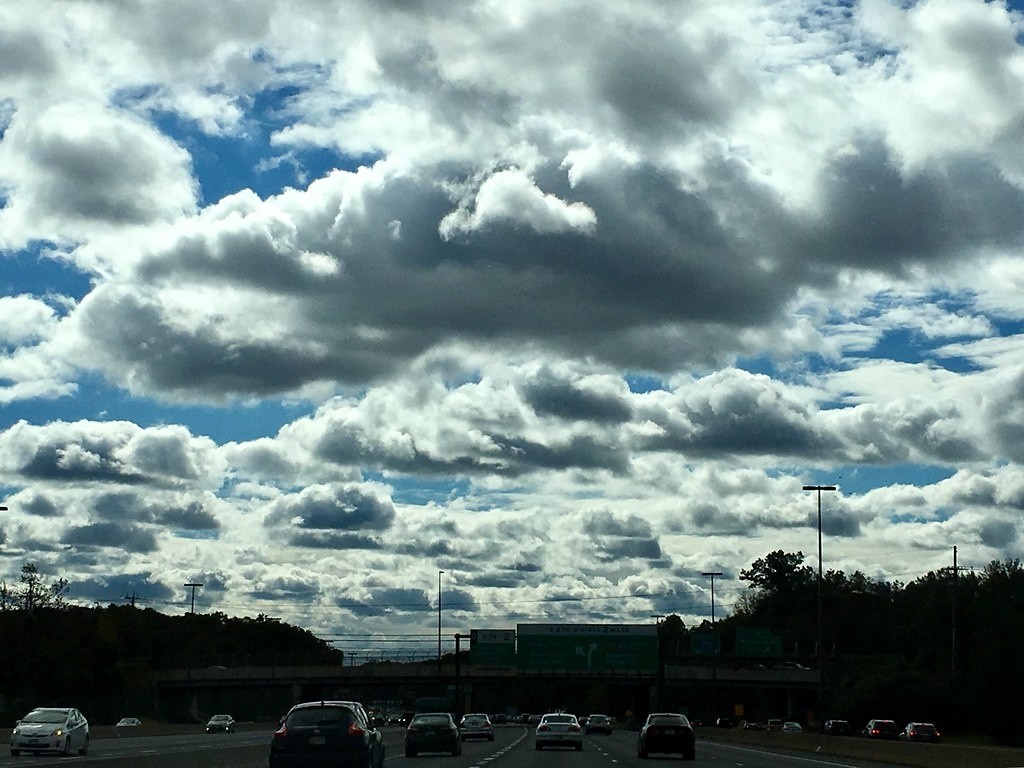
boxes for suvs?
[904,722,940,742]
[861,719,899,740]
[823,720,852,736]
[268,701,387,768]
[584,714,612,735]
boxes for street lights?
[438,570,444,661]
[803,486,837,736]
[651,614,665,625]
[702,572,724,702]
[184,583,203,696]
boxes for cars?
[404,712,461,757]
[459,712,494,742]
[366,703,405,727]
[487,712,589,726]
[690,718,803,733]
[637,712,696,761]
[116,717,142,727]
[535,713,583,753]
[9,707,89,756]
[205,715,236,734]
